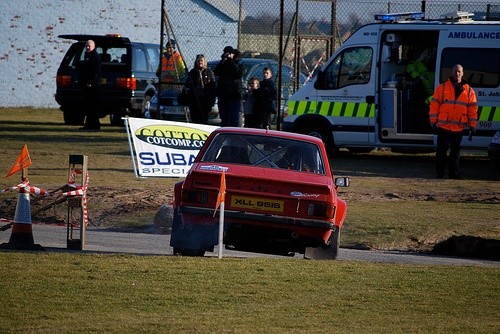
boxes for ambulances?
[279,11,500,180]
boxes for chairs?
[215,146,251,165]
[98,54,111,62]
[275,146,315,172]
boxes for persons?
[258,66,279,129]
[156,37,186,85]
[243,77,268,129]
[183,54,218,125]
[428,64,480,180]
[405,48,434,96]
[76,39,101,132]
[214,45,244,127]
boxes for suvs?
[54,32,187,127]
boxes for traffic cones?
[0,176,46,251]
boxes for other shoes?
[448,173,464,179]
[78,125,101,132]
[435,174,448,179]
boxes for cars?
[169,126,351,261]
[147,58,308,128]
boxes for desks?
[382,88,398,131]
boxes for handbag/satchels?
[176,88,190,107]
